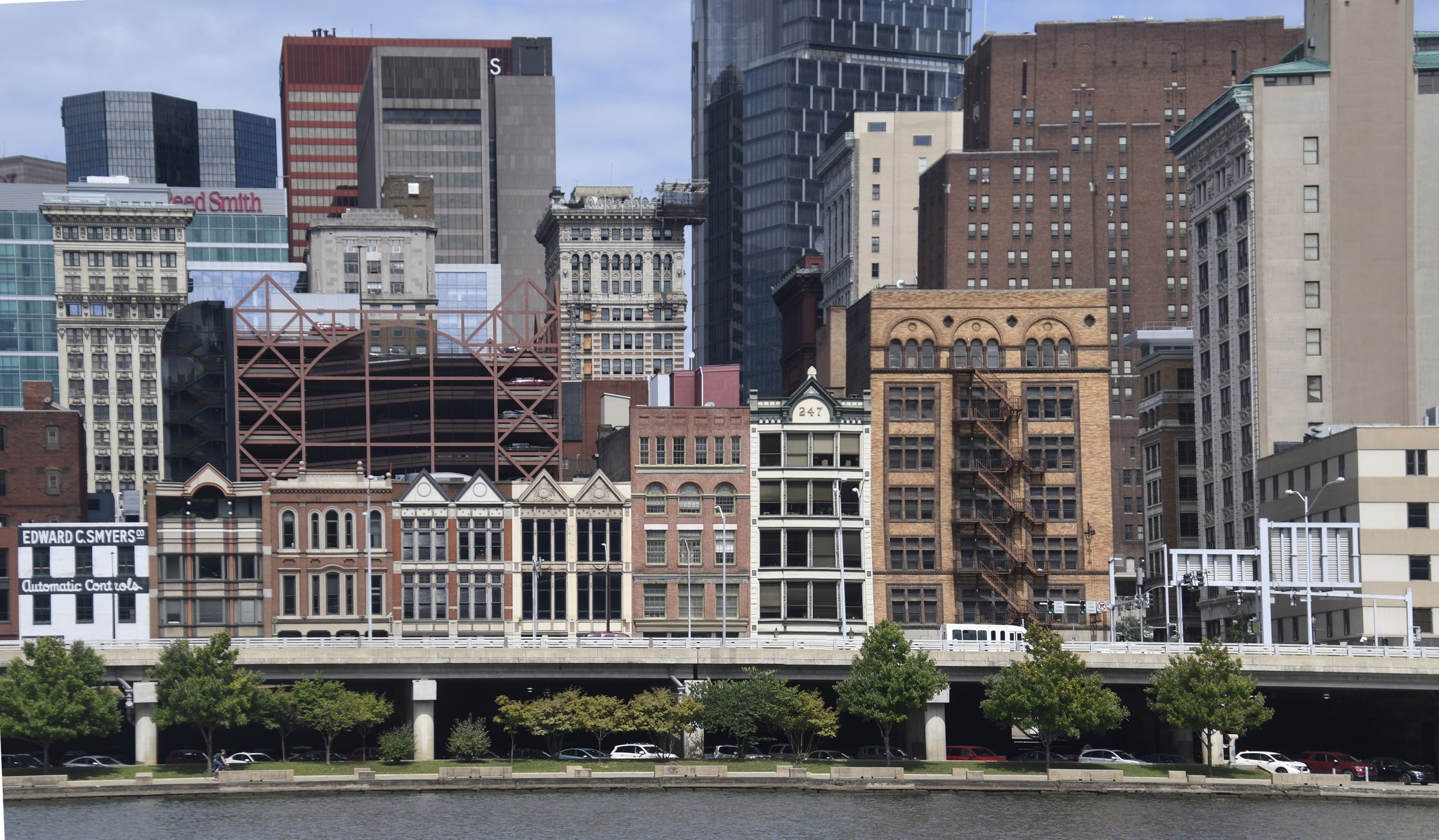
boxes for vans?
[940,622,1031,651]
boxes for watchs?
[226,763,228,765]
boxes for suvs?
[581,631,648,649]
[610,742,679,759]
[704,744,1433,788]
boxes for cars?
[1,754,37,769]
[288,746,312,759]
[225,752,277,764]
[13,754,54,768]
[348,747,388,762]
[452,748,612,762]
[60,753,85,765]
[62,755,128,767]
[165,750,209,763]
[289,750,348,761]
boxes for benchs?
[1271,773,1351,787]
[654,765,727,777]
[438,766,512,778]
[2,775,68,787]
[1047,769,1124,781]
[218,769,294,782]
[830,767,904,779]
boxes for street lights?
[111,552,115,640]
[600,506,728,647]
[836,475,850,648]
[1139,586,1174,643]
[1284,477,1348,648]
[367,475,379,639]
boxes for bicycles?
[204,764,235,781]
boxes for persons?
[213,749,229,774]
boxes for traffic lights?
[1184,570,1205,591]
[1145,592,1154,607]
[1079,600,1086,615]
[1047,600,1054,614]
